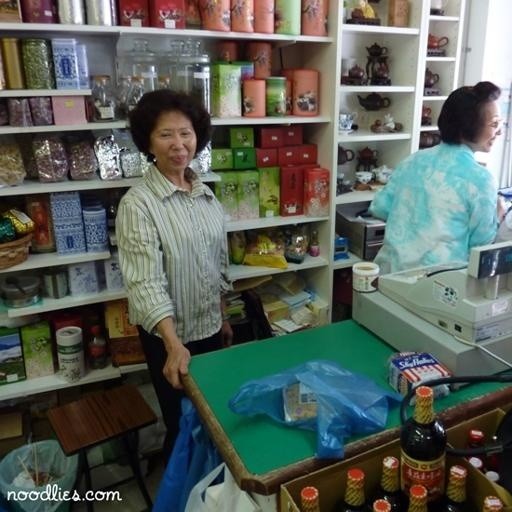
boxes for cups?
[352,171,374,184]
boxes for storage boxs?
[277,424,510,511]
[444,406,511,511]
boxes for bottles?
[89,326,108,369]
[22,37,54,91]
[87,74,176,121]
[56,327,87,383]
[275,385,506,512]
[309,230,320,257]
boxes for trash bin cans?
[0,440,81,512]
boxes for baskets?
[0,232,33,269]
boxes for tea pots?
[425,69,439,88]
[339,108,358,129]
[427,33,449,50]
[363,42,387,56]
[358,147,379,159]
[371,166,394,184]
[338,144,354,165]
[421,106,433,117]
[348,64,388,78]
[420,133,440,150]
[355,92,390,110]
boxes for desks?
[175,316,512,499]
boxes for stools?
[46,380,161,511]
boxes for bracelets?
[221,311,231,321]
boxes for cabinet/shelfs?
[416,1,469,153]
[1,14,129,405]
[303,2,424,326]
[112,2,340,373]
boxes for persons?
[367,81,503,277]
[113,85,234,459]
